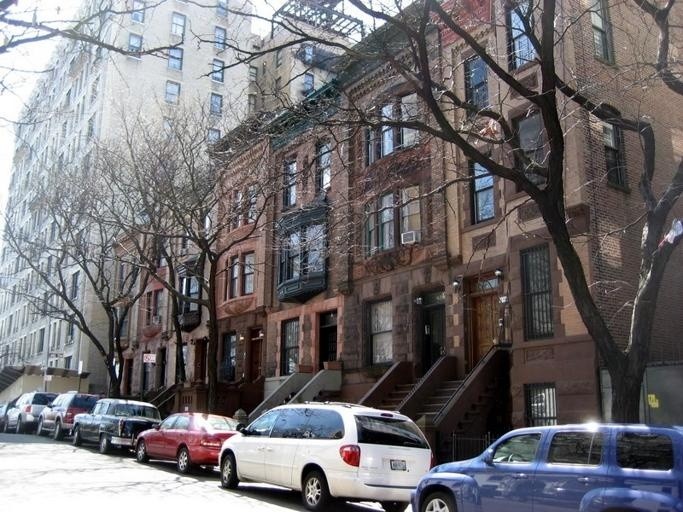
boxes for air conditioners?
[400,231,421,245]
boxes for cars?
[71,398,163,456]
[134,411,242,475]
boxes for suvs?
[217,402,435,511]
[37,391,106,441]
[412,415,682,511]
[1,392,58,434]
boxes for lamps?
[452,280,461,291]
[494,268,504,280]
